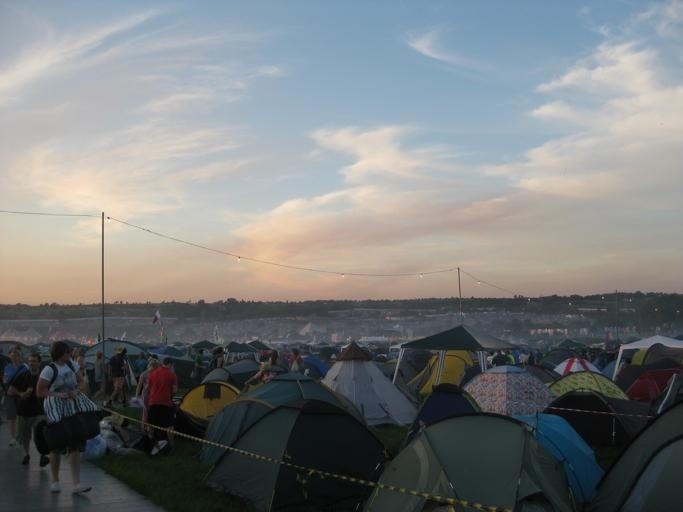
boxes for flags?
[151,310,160,324]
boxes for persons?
[0,340,93,495]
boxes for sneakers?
[150,440,167,456]
[71,482,93,495]
[51,481,62,493]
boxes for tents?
[84,323,683,511]
